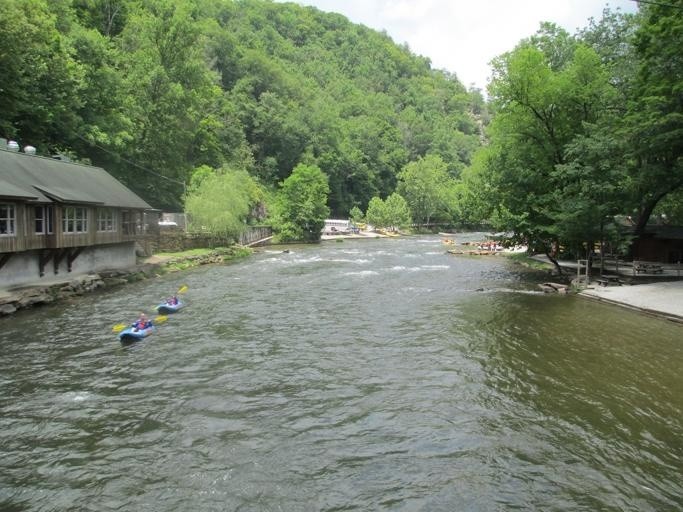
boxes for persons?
[480,240,496,251]
[167,295,177,305]
[131,313,152,333]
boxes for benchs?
[596,280,607,287]
[614,279,626,286]
[636,262,664,274]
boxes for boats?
[112,316,157,340]
[442,236,507,259]
[157,298,186,312]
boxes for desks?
[601,274,619,286]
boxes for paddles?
[112,315,166,339]
[175,286,185,296]
